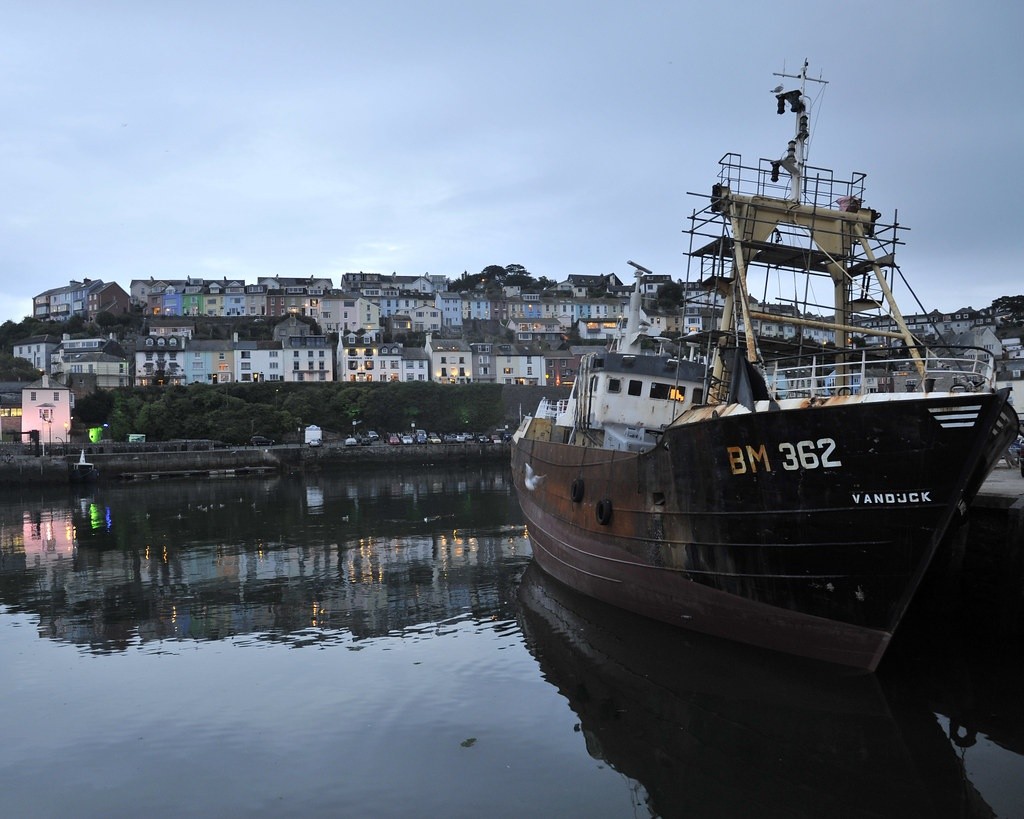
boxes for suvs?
[248,436,276,446]
[366,431,380,439]
[446,434,466,442]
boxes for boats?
[511,58,1018,673]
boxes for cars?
[346,438,357,445]
[310,438,323,447]
[427,436,441,444]
[388,436,400,445]
[401,436,413,445]
[414,430,426,444]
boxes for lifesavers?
[594,498,613,525]
[569,479,584,503]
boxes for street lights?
[64,422,69,455]
